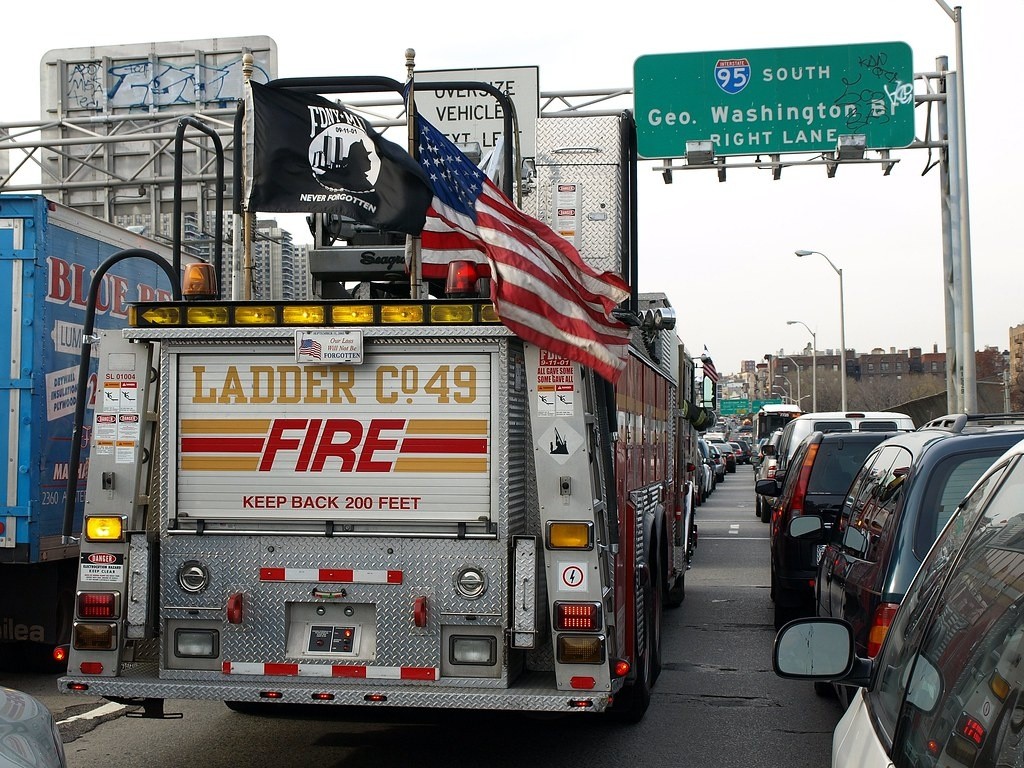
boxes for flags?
[704,347,719,383]
[244,80,434,236]
[412,110,629,384]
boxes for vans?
[761,411,916,511]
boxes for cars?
[696,415,784,523]
[772,436,1023,768]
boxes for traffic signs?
[634,41,916,164]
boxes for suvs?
[755,425,917,633]
[787,411,1024,712]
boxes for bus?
[751,403,803,472]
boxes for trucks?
[0,189,211,682]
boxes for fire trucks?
[58,46,719,728]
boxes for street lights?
[792,249,848,414]
[771,385,810,405]
[786,320,817,413]
[774,374,793,405]
[778,356,800,409]
